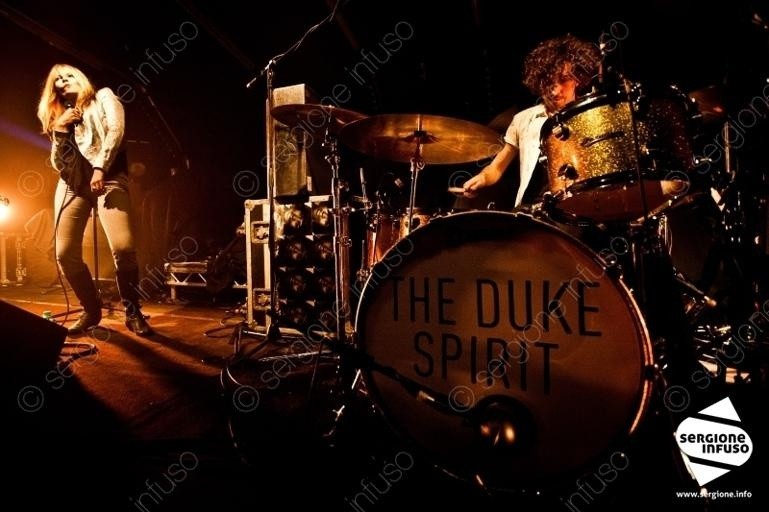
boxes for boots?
[66,264,102,334]
[115,268,151,335]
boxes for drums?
[539,85,701,224]
[356,208,669,477]
[360,209,446,282]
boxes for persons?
[462,36,603,208]
[37,63,154,337]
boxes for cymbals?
[337,115,505,165]
[272,105,366,136]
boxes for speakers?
[0,298,68,416]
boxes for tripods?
[202,62,385,458]
[42,197,152,339]
